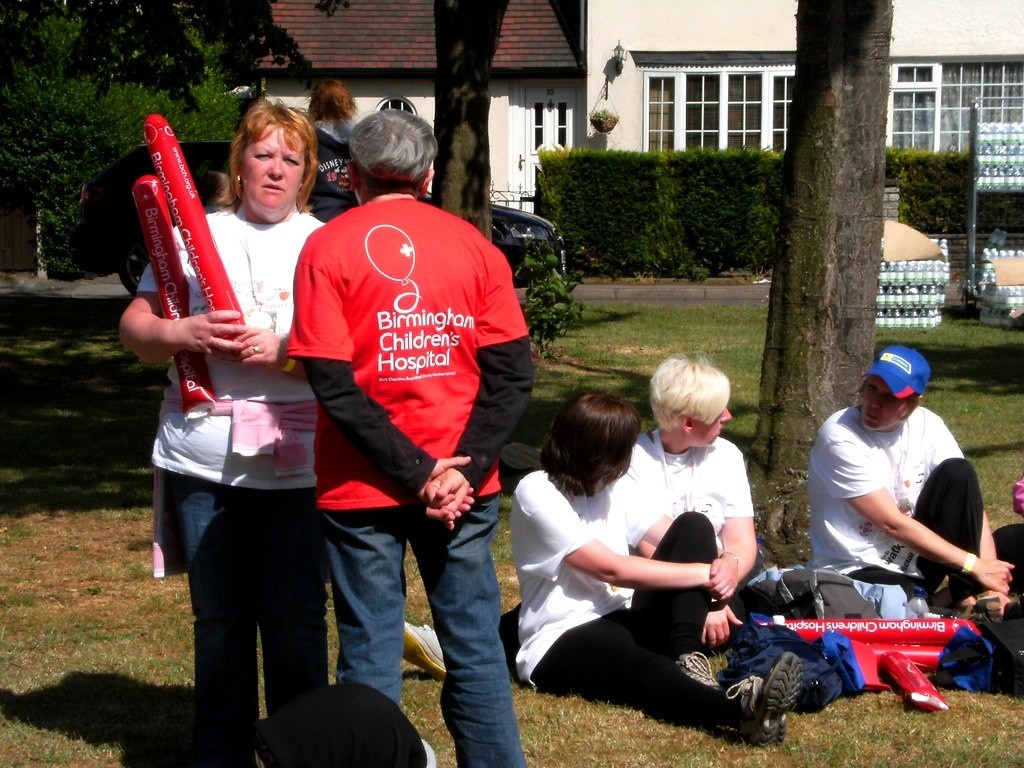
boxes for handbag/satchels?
[715,562,1024,714]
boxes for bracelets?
[719,552,739,566]
[962,553,977,574]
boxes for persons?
[121,81,535,768]
[807,343,1017,619]
[402,343,804,749]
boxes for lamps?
[613,39,628,74]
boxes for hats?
[861,345,931,399]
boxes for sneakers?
[402,621,447,681]
[726,652,804,746]
[677,650,725,693]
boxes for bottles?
[743,536,770,587]
[906,587,929,619]
[875,238,951,328]
[974,121,1024,193]
[977,248,1024,328]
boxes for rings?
[253,345,261,354]
[431,482,441,490]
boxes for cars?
[70,139,563,299]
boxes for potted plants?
[590,110,619,132]
